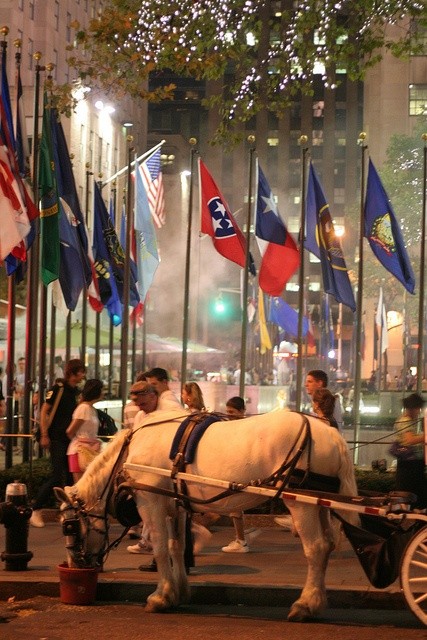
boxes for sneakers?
[221,540,249,553]
[193,526,213,555]
[127,542,154,555]
[30,511,46,528]
[275,515,292,530]
[184,558,191,574]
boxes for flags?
[302,158,357,313]
[317,293,335,356]
[248,281,272,355]
[263,291,308,338]
[253,157,300,297]
[197,156,246,269]
[364,155,416,296]
[353,303,389,361]
[2,38,165,330]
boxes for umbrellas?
[38,320,133,360]
[128,332,226,354]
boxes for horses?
[53,411,359,623]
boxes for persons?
[139,510,195,575]
[30,359,86,527]
[129,381,183,426]
[122,373,148,429]
[305,370,328,395]
[221,511,250,553]
[0,356,25,455]
[54,356,64,380]
[33,391,42,457]
[226,396,245,420]
[181,382,206,410]
[225,362,274,386]
[393,394,427,514]
[312,388,339,430]
[127,524,142,539]
[126,517,213,556]
[66,379,105,484]
[143,367,180,403]
[369,369,417,393]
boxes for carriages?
[54,412,426,624]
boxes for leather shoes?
[138,558,158,571]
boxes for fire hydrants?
[1,480,32,569]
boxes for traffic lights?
[211,298,231,321]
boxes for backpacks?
[33,382,64,449]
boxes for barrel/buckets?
[57,559,99,607]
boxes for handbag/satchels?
[389,439,417,458]
[73,434,102,472]
